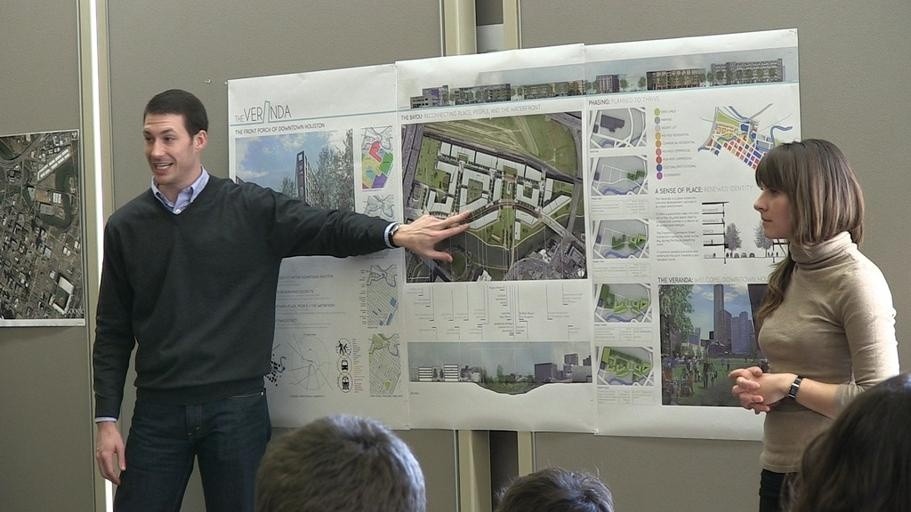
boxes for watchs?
[389,223,399,250]
[788,374,805,402]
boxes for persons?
[781,372,911,511]
[254,417,426,512]
[728,139,898,512]
[92,90,469,512]
[499,468,614,512]
